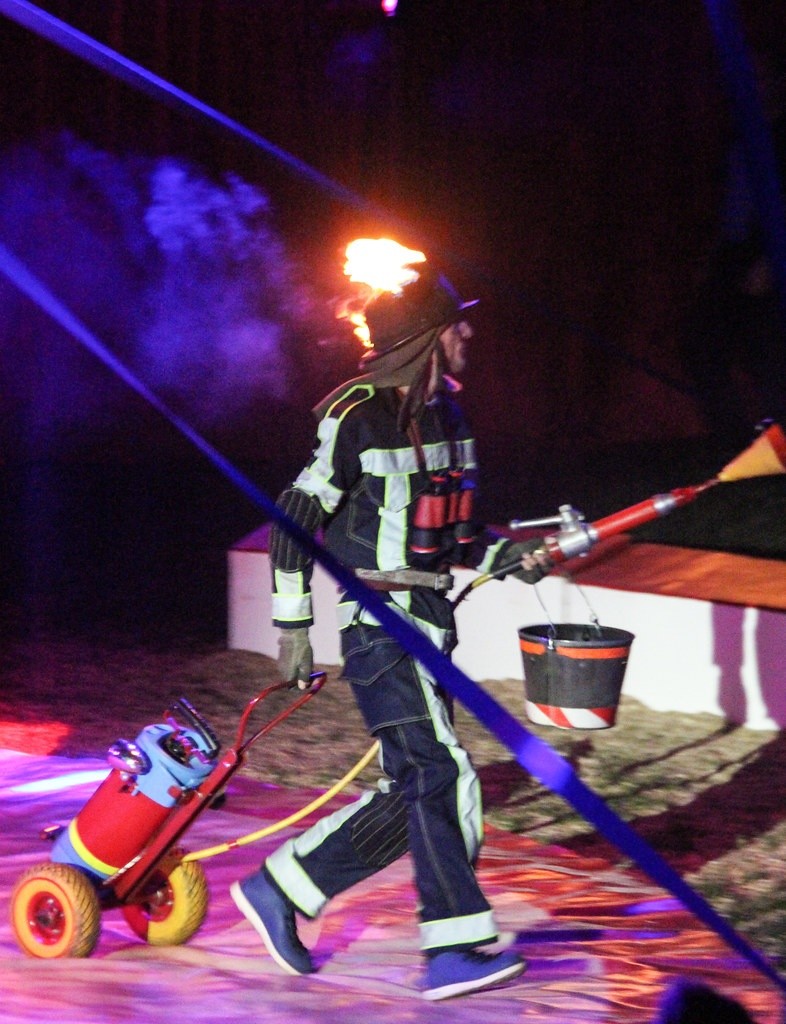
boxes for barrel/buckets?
[519,563,635,730]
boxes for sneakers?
[418,947,527,1000]
[230,869,312,975]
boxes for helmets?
[362,272,480,362]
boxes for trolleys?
[7,670,329,961]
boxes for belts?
[354,568,453,592]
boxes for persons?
[232,266,555,1000]
